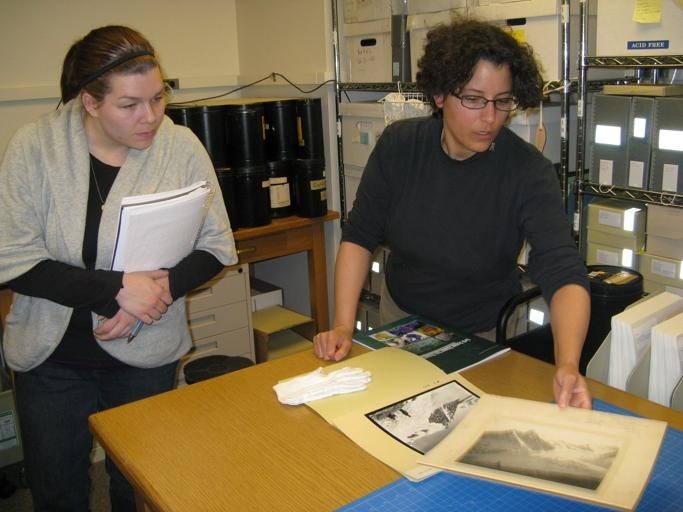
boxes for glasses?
[448,91,519,112]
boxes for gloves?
[271,365,372,406]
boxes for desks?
[231,210,339,335]
[83,323,681,511]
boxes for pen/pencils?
[127,320,144,344]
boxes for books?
[350,314,513,377]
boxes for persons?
[311,19,595,411]
[1,24,241,510]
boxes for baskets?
[377,92,433,127]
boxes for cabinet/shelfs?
[172,261,258,388]
